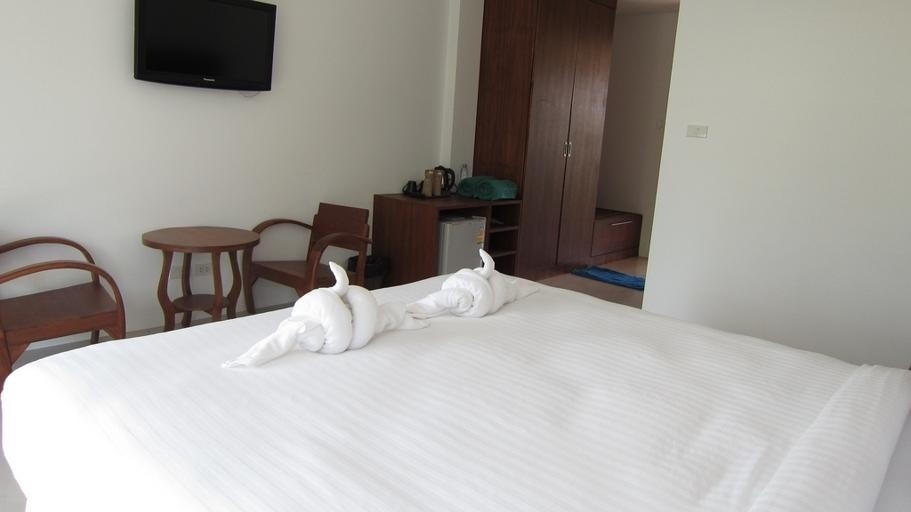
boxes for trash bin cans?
[348,255,384,290]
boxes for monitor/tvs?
[133,1,276,93]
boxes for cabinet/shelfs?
[473,0,617,282]
[591,207,643,266]
[373,193,523,289]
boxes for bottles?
[460,164,469,180]
[424,170,433,197]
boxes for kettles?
[433,166,455,192]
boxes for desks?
[142,225,260,331]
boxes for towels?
[458,175,497,198]
[479,180,517,201]
[223,261,431,370]
[405,246,539,319]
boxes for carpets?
[570,266,645,290]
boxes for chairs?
[1,236,126,389]
[243,202,374,314]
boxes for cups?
[402,181,417,192]
[416,181,424,192]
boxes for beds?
[3,272,910,511]
